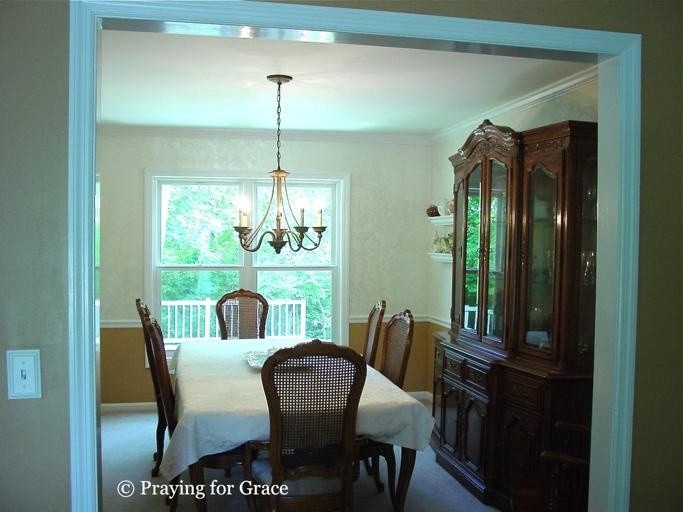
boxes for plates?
[246,351,314,371]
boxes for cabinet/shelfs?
[440,352,503,480]
[515,121,598,376]
[495,369,592,512]
[447,118,518,361]
[425,215,454,263]
[432,338,445,419]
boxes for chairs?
[135,297,231,477]
[362,300,387,475]
[242,339,367,512]
[142,303,259,511]
[352,308,414,509]
[216,289,268,340]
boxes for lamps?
[233,75,328,253]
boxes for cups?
[547,248,553,277]
[580,249,596,283]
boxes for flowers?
[429,202,437,207]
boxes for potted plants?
[425,205,439,217]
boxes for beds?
[157,338,436,511]
